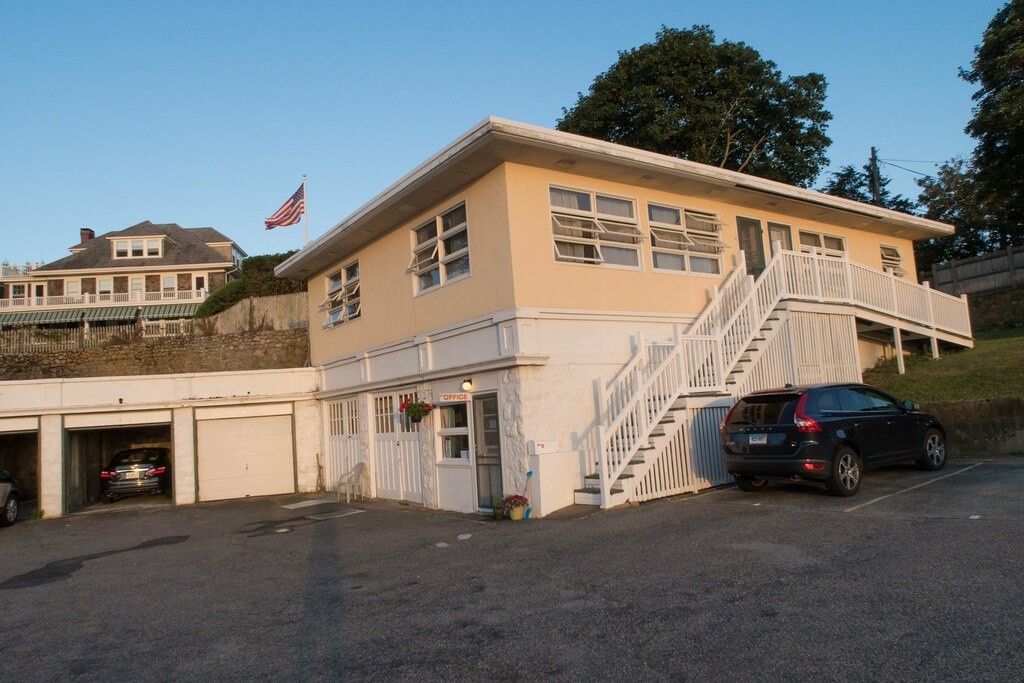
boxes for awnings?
[0,304,199,326]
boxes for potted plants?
[493,497,505,519]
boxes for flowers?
[400,399,432,416]
[504,495,528,510]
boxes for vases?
[411,415,422,422]
[509,506,524,520]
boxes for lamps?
[462,379,472,390]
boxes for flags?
[265,183,305,230]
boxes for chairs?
[337,462,365,503]
[122,456,159,462]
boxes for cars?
[0,471,22,526]
[98,447,173,504]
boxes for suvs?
[718,381,948,496]
